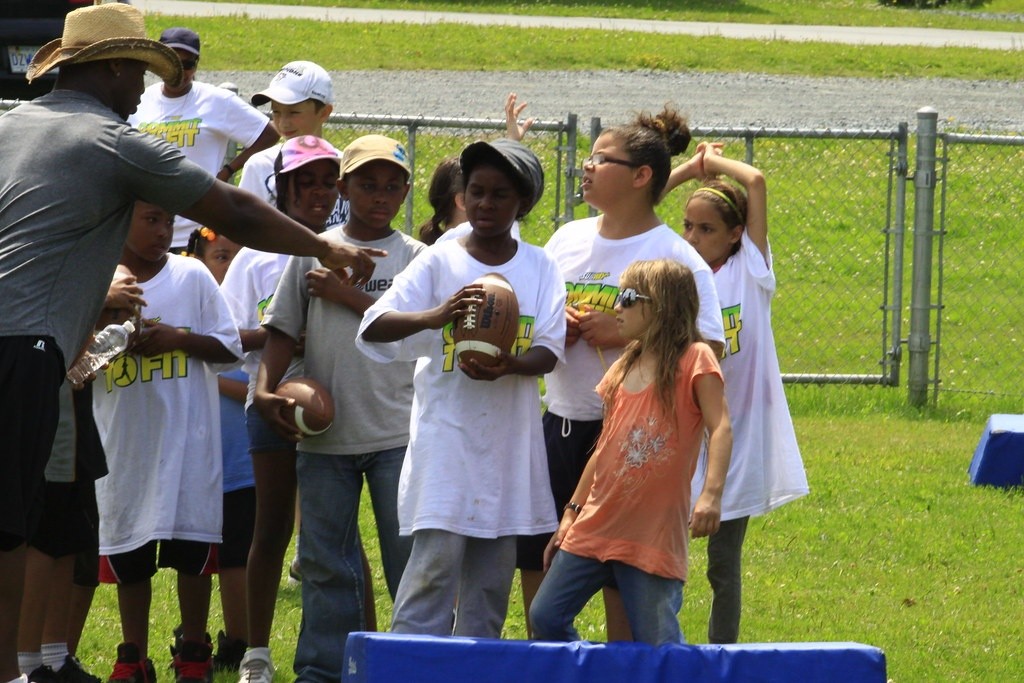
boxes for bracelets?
[564,502,582,514]
[224,164,236,174]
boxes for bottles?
[67,321,135,384]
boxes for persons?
[515,108,727,642]
[419,92,534,246]
[655,141,810,643]
[527,258,733,643]
[356,141,567,640]
[239,60,350,229]
[253,135,428,683]
[18,135,349,683]
[0,3,387,683]
[126,29,281,181]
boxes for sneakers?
[28,664,63,683]
[238,647,274,683]
[56,654,102,683]
[169,633,212,683]
[109,642,157,683]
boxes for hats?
[339,135,410,184]
[274,135,342,176]
[25,2,182,87]
[460,138,544,216]
[159,28,200,56]
[251,61,333,109]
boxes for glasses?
[181,56,198,70]
[618,288,652,307]
[582,152,656,178]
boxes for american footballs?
[276,377,335,437]
[454,272,522,372]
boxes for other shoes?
[212,630,246,671]
